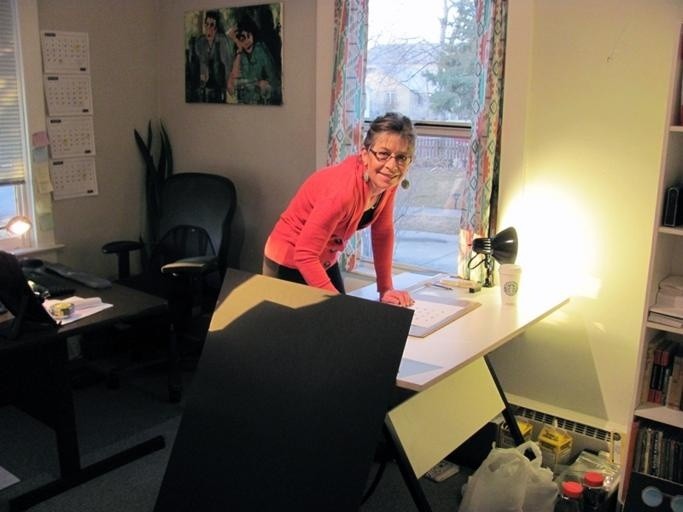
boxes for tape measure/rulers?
[50,302,75,316]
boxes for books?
[628,311,683,485]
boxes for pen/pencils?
[432,284,452,290]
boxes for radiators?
[489,388,627,461]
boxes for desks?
[322,259,574,399]
[2,247,172,512]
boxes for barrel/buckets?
[554,472,608,512]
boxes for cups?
[497,262,524,306]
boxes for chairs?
[380,354,558,510]
[145,269,418,512]
[93,170,243,410]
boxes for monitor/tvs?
[0,250,57,341]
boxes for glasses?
[370,149,412,167]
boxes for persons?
[194,10,232,103]
[259,109,417,308]
[224,15,280,105]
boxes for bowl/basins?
[48,301,76,319]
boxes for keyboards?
[23,268,75,299]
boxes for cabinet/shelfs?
[611,14,683,511]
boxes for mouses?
[17,257,43,268]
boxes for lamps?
[1,214,38,241]
[464,223,523,296]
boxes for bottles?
[552,482,583,512]
[581,472,606,511]
